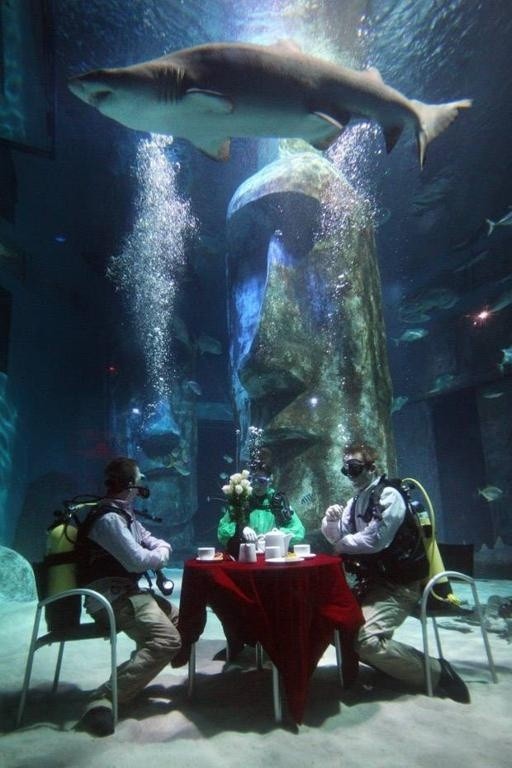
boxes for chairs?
[12,556,126,732]
[404,542,500,698]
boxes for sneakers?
[79,688,114,727]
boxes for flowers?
[214,463,257,515]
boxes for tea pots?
[256,528,293,556]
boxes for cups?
[293,544,311,557]
[197,546,216,559]
[239,543,257,563]
[265,546,281,559]
[255,540,266,553]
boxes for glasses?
[250,474,273,487]
[340,459,376,477]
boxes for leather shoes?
[435,656,471,705]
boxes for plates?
[196,556,224,562]
[291,553,317,560]
[266,557,304,564]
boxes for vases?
[224,510,252,556]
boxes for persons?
[209,460,306,662]
[71,457,185,731]
[316,438,473,705]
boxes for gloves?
[325,504,344,521]
[242,526,258,542]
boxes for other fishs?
[172,315,191,349]
[184,379,204,398]
[391,395,409,416]
[484,210,512,236]
[192,331,223,358]
[475,485,504,503]
[392,327,512,404]
[165,452,192,478]
